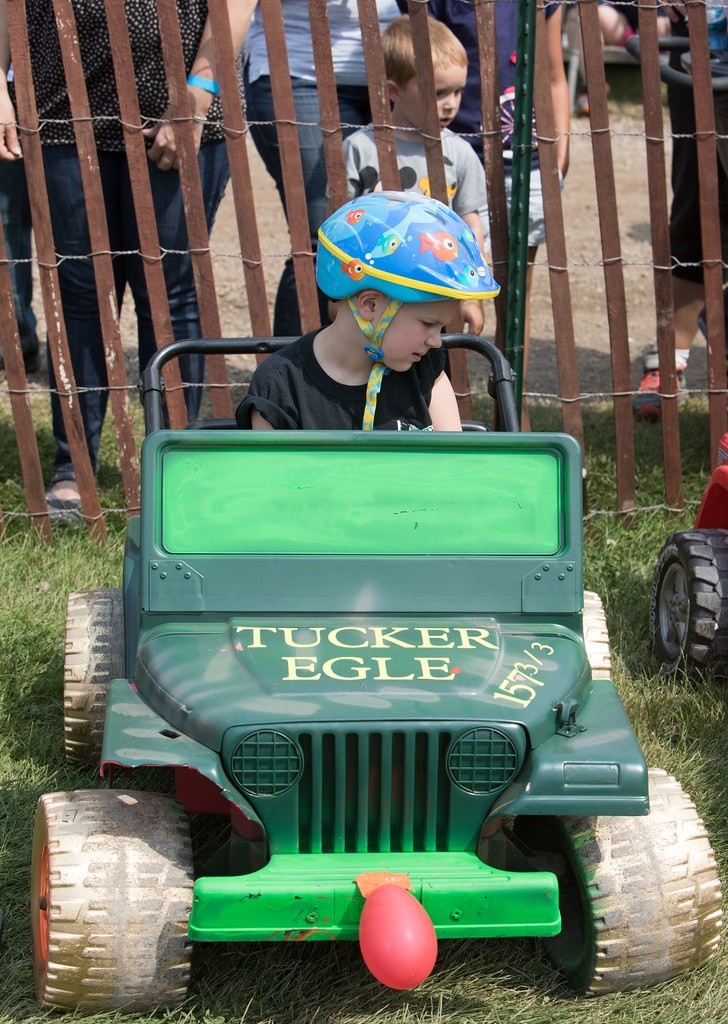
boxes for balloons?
[359,883,439,990]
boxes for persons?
[598,2,727,421]
[251,0,407,342]
[235,188,503,432]
[1,1,257,516]
[396,0,571,432]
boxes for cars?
[31,336,722,1024]
[651,465,726,671]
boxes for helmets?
[315,191,501,302]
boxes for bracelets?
[186,72,220,95]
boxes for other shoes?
[45,472,85,523]
[633,353,688,420]
